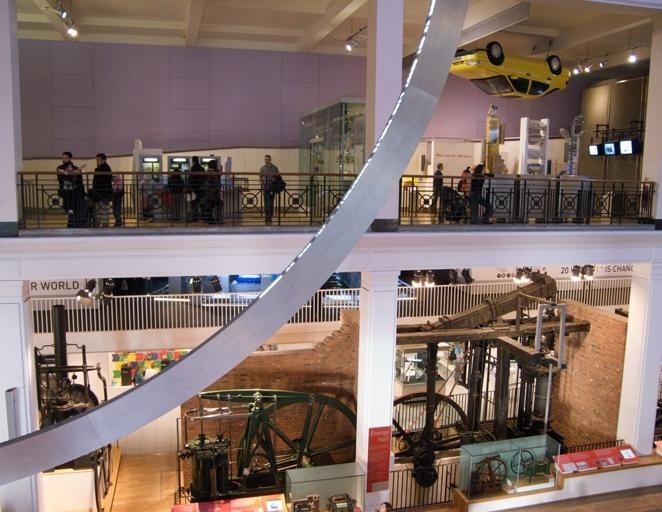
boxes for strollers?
[441,184,470,225]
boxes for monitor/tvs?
[587,143,604,157]
[619,139,638,155]
[293,499,319,512]
[604,141,618,155]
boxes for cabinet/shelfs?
[517,117,549,175]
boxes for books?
[616,444,642,465]
[567,451,599,472]
[553,454,577,475]
[593,447,624,469]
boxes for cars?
[448,39,572,101]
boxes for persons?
[431,162,444,212]
[258,154,280,226]
[139,153,237,225]
[112,174,124,226]
[54,150,92,228]
[62,161,81,215]
[88,152,114,228]
[377,502,394,512]
[461,165,473,200]
[468,163,496,224]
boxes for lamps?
[411,264,596,288]
[55,9,79,39]
[74,279,97,304]
[572,49,638,75]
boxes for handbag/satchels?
[270,175,286,193]
